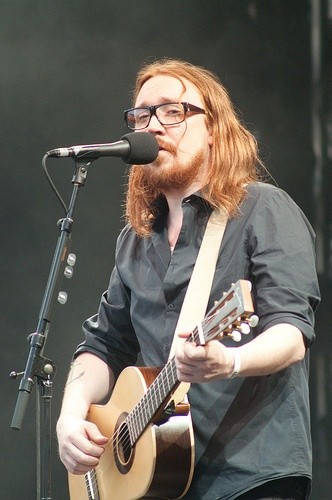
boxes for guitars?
[65,279,259,500]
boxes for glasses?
[123,102,206,131]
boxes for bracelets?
[225,346,241,380]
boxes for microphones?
[46,132,158,165]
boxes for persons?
[54,61,324,500]
